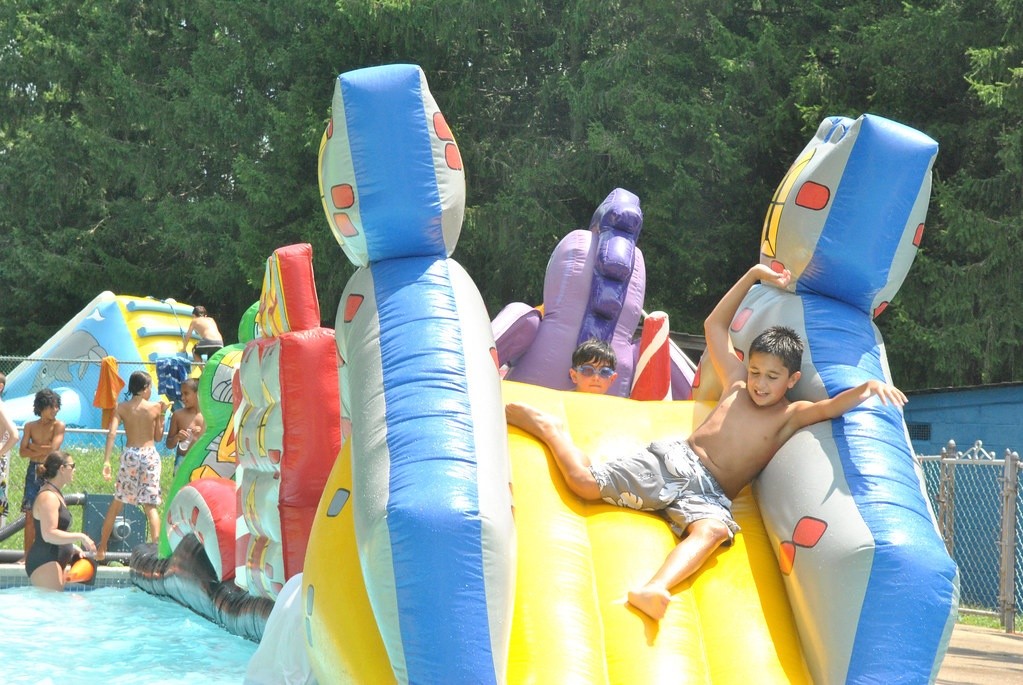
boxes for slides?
[387,375,846,685]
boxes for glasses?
[64,462,75,469]
[573,365,614,378]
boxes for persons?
[505,263,908,619]
[0,369,207,593]
[178,305,223,373]
